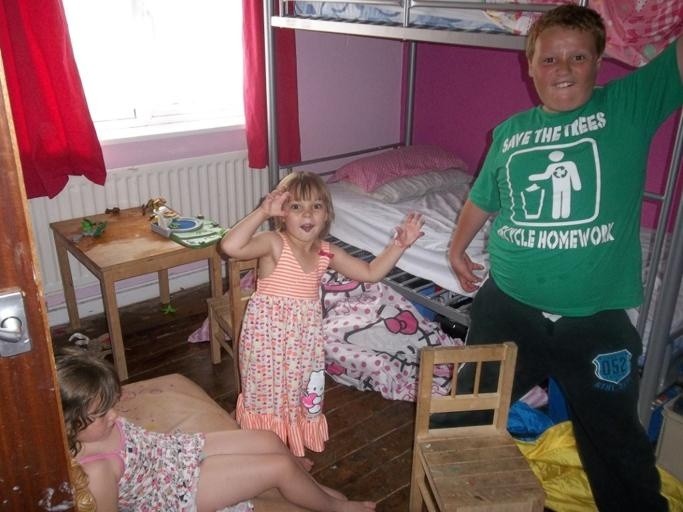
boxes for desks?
[49,205,224,381]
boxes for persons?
[54,338,378,511]
[427,4,683,512]
[219,171,425,471]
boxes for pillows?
[323,143,470,192]
[332,167,478,204]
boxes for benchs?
[61,373,313,512]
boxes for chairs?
[206,257,259,398]
[407,343,548,512]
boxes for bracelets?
[392,235,411,250]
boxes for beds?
[261,0,682,444]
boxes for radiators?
[28,149,290,312]
